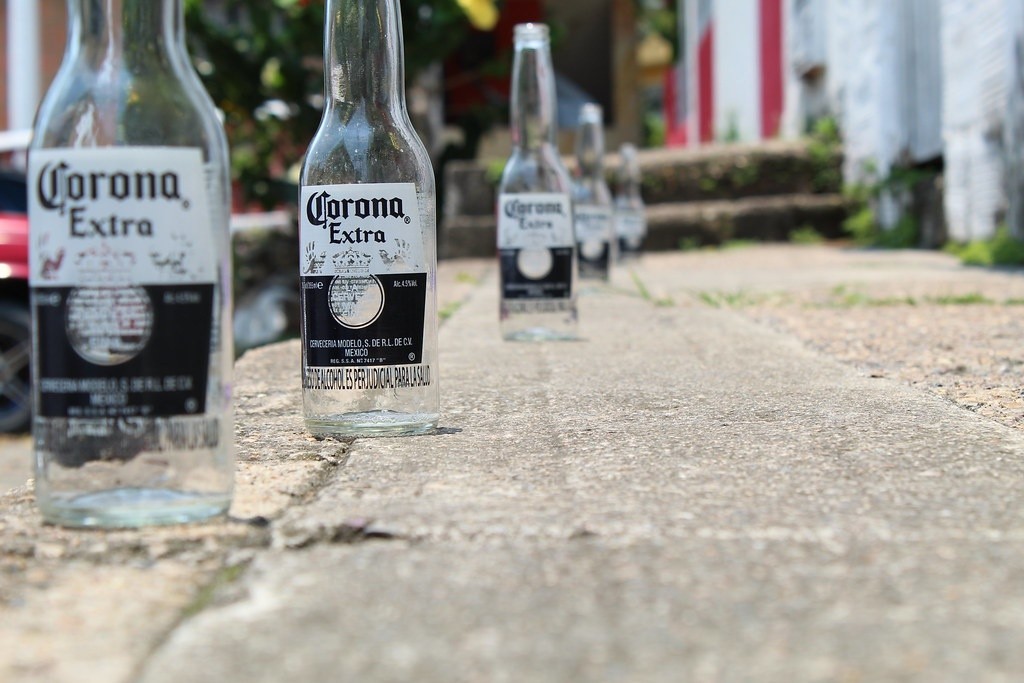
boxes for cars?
[240,59,602,212]
[0,132,302,435]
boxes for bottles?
[615,146,648,278]
[571,103,624,287]
[498,23,576,340]
[29,0,235,529]
[296,0,439,435]
